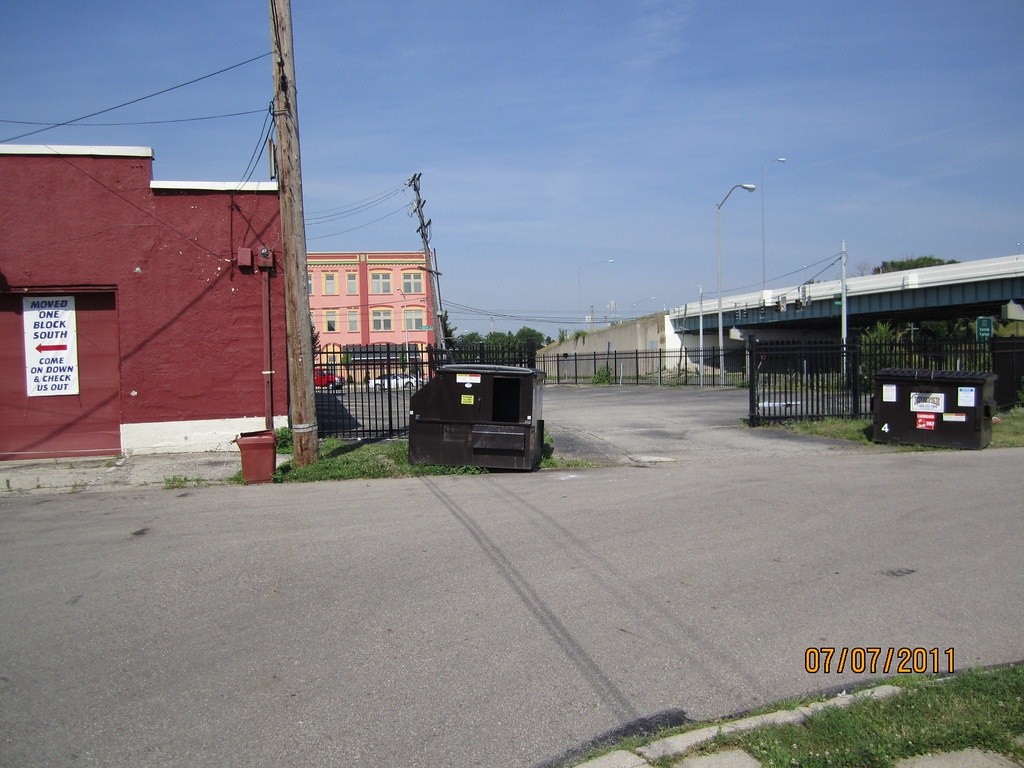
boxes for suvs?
[314,368,345,390]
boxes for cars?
[367,373,428,392]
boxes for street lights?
[761,157,786,291]
[578,259,614,331]
[715,184,757,386]
[642,297,656,315]
[396,287,409,373]
[419,298,437,348]
[631,303,637,320]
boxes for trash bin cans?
[408,364,548,470]
[872,368,999,450]
[233,429,277,485]
